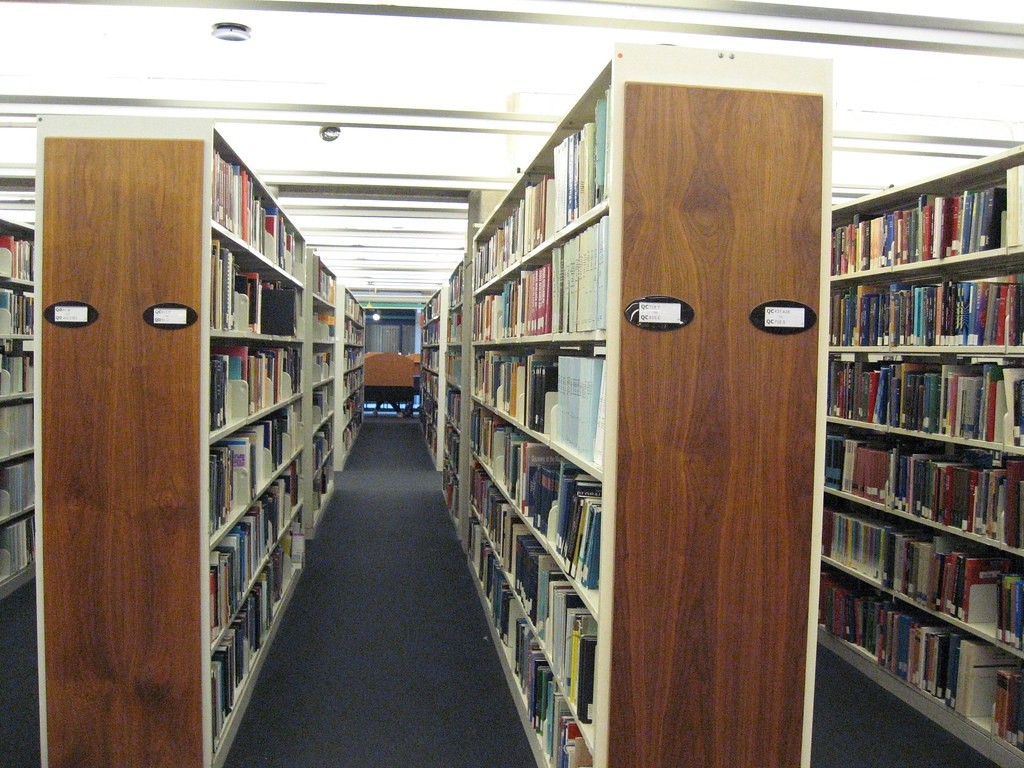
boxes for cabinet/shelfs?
[1,42,1024,766]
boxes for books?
[817,163,1023,757]
[421,86,609,768]
[1,235,36,582]
[209,148,363,762]
